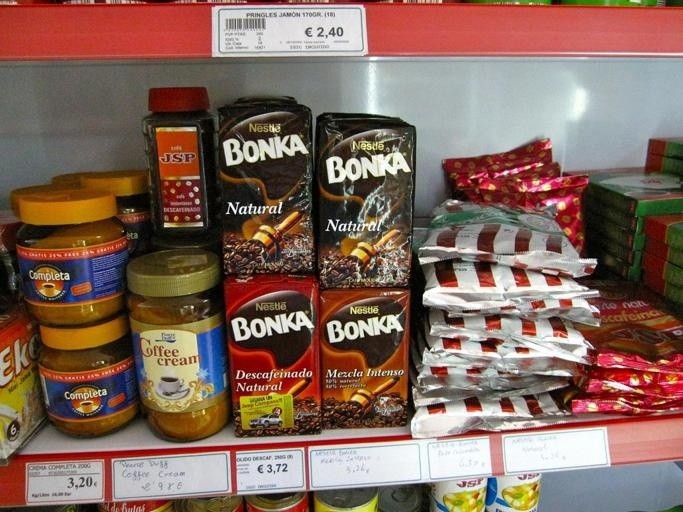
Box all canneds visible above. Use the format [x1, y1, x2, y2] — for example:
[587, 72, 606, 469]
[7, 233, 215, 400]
[314, 488, 379, 512]
[376, 483, 431, 512]
[431, 478, 489, 512]
[186, 495, 244, 512]
[11, 170, 233, 444]
[245, 490, 309, 512]
[486, 474, 542, 512]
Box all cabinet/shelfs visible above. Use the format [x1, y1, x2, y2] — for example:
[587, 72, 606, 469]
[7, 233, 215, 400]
[0, 0, 683, 511]
